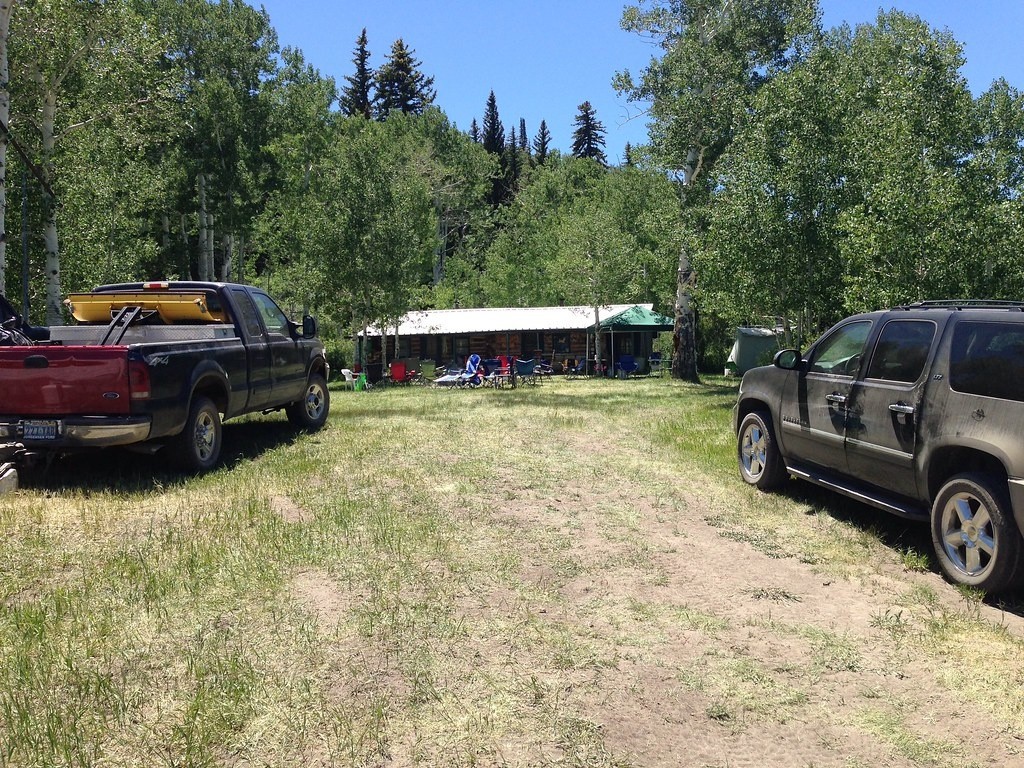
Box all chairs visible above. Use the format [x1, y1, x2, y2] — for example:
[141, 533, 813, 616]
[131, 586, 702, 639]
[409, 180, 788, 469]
[404, 351, 665, 388]
[886, 343, 931, 384]
[341, 369, 359, 391]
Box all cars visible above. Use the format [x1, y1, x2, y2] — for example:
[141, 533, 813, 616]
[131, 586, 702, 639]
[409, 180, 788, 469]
[731, 298, 1024, 596]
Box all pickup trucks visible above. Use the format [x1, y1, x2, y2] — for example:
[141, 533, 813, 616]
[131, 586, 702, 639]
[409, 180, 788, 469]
[0, 272, 334, 481]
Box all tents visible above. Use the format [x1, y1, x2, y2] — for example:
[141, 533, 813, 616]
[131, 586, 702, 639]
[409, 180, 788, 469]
[586, 305, 675, 380]
